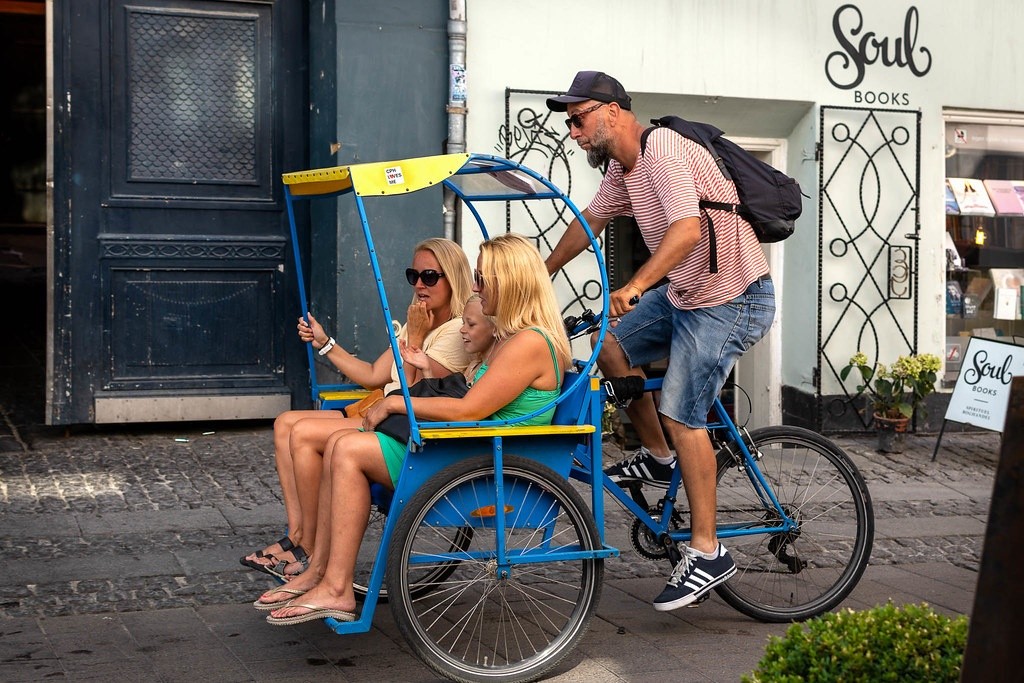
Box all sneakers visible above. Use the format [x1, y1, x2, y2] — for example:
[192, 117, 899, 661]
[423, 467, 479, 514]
[653, 542, 738, 612]
[602, 445, 683, 489]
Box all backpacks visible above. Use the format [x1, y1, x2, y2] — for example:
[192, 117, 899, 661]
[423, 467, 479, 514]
[640, 114, 811, 273]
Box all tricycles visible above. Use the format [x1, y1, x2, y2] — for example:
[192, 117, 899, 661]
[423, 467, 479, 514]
[273, 152, 875, 683]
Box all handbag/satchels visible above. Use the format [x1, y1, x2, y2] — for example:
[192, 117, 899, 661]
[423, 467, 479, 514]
[374, 372, 470, 445]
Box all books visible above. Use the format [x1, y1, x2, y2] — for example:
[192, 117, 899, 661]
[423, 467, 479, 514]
[946, 269, 1024, 321]
[945, 174, 1024, 223]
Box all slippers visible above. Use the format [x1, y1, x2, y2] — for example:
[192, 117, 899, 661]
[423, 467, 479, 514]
[266, 599, 356, 625]
[253, 585, 307, 610]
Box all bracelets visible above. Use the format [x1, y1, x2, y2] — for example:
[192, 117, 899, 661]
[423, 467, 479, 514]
[319, 337, 335, 355]
[318, 338, 330, 351]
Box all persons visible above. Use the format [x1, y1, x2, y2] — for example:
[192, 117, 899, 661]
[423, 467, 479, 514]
[238, 238, 474, 574]
[544, 72, 774, 611]
[253, 235, 569, 626]
[266, 294, 497, 583]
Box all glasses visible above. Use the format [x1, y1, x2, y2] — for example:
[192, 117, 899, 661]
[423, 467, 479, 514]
[565, 103, 604, 130]
[406, 268, 445, 287]
[474, 269, 497, 286]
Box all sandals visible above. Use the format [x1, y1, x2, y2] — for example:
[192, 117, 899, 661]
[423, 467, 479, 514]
[281, 576, 290, 584]
[248, 546, 308, 574]
[264, 556, 309, 578]
[240, 536, 295, 567]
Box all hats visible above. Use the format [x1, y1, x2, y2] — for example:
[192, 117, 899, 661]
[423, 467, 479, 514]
[546, 71, 632, 112]
